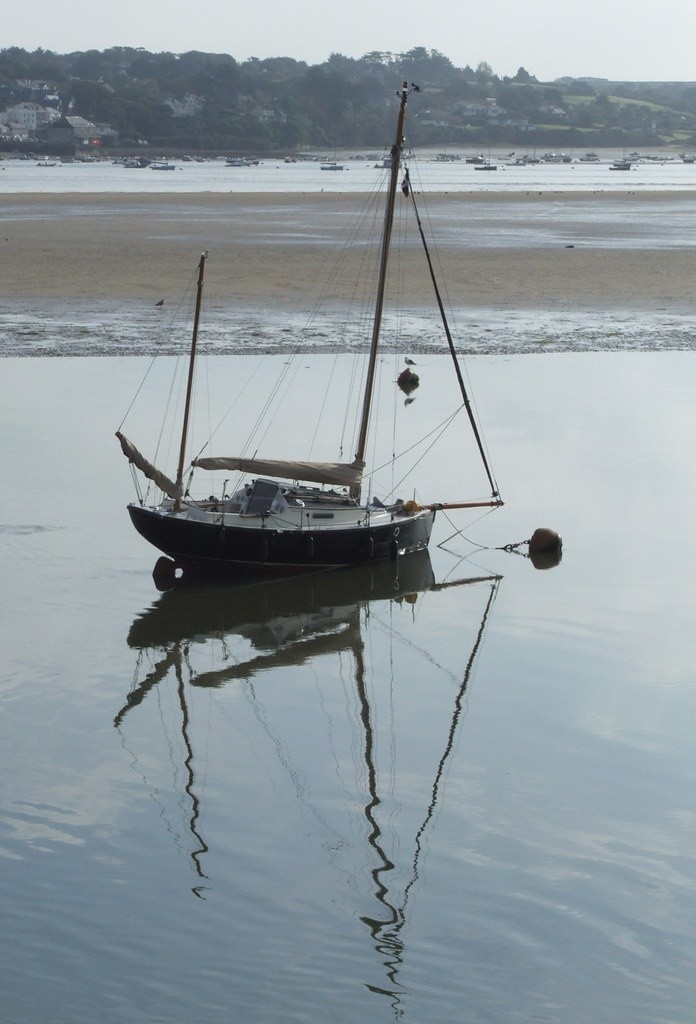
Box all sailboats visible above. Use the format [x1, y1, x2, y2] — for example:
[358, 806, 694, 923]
[113, 547, 505, 924]
[112, 74, 504, 566]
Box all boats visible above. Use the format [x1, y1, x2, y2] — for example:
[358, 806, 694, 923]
[36, 145, 696, 172]
